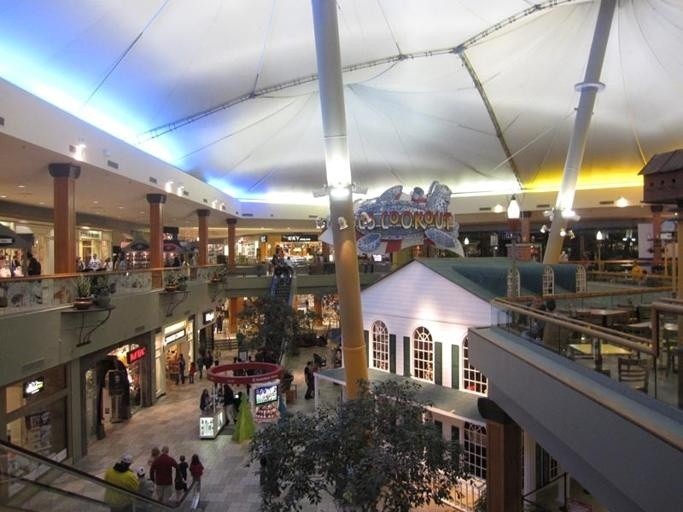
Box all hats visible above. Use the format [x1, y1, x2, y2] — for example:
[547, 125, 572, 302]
[137, 466, 146, 475]
[122, 455, 133, 463]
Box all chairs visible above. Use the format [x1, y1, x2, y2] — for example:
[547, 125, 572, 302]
[518, 303, 680, 394]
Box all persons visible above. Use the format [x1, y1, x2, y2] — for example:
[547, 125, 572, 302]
[270, 244, 294, 285]
[632, 260, 642, 281]
[304, 345, 343, 401]
[369, 254, 375, 271]
[104, 445, 205, 512]
[11, 251, 43, 278]
[179, 349, 254, 425]
[518, 297, 545, 336]
[642, 270, 648, 285]
[172, 247, 202, 278]
[76, 250, 127, 276]
[536, 300, 569, 336]
[364, 255, 369, 272]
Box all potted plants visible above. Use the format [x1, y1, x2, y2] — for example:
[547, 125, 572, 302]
[164, 272, 189, 291]
[72, 275, 111, 310]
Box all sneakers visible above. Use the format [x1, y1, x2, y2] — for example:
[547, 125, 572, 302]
[304, 394, 312, 399]
